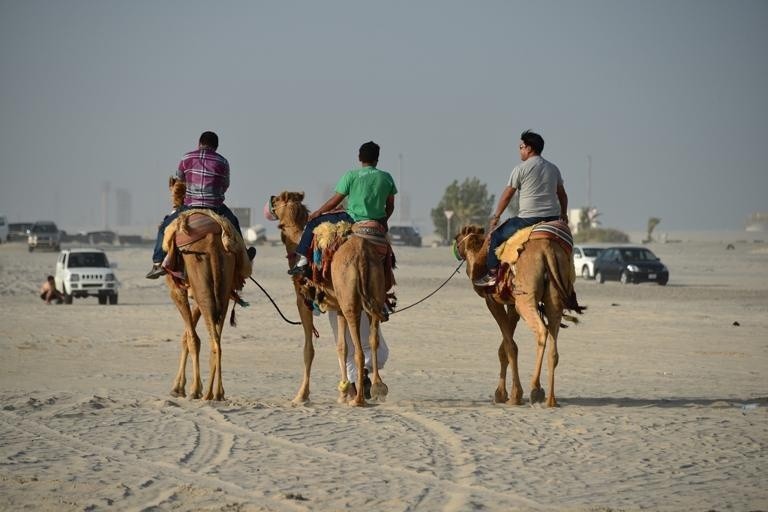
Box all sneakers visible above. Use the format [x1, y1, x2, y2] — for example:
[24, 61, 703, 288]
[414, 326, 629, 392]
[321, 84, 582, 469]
[146, 263, 167, 279]
[474, 270, 496, 286]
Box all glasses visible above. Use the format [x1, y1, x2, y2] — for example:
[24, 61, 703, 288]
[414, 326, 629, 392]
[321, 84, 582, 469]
[520, 145, 527, 150]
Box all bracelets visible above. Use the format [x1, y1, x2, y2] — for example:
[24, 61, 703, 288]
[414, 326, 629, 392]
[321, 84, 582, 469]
[493, 214, 501, 221]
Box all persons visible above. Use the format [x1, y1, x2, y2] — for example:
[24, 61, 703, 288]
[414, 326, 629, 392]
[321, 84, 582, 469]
[482, 131, 570, 275]
[38, 273, 66, 305]
[287, 141, 399, 276]
[146, 130, 256, 280]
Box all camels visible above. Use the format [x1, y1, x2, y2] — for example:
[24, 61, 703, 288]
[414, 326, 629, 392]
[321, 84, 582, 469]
[262, 190, 397, 407]
[165, 175, 250, 405]
[454, 220, 587, 409]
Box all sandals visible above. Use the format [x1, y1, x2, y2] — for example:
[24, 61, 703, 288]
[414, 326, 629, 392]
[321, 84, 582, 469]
[288, 264, 309, 275]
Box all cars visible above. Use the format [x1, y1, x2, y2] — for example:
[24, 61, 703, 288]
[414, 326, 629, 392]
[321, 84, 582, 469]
[573, 245, 669, 286]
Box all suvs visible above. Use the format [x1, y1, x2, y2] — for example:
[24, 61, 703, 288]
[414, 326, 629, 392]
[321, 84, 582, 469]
[387, 226, 421, 247]
[25, 221, 61, 253]
[53, 248, 118, 305]
